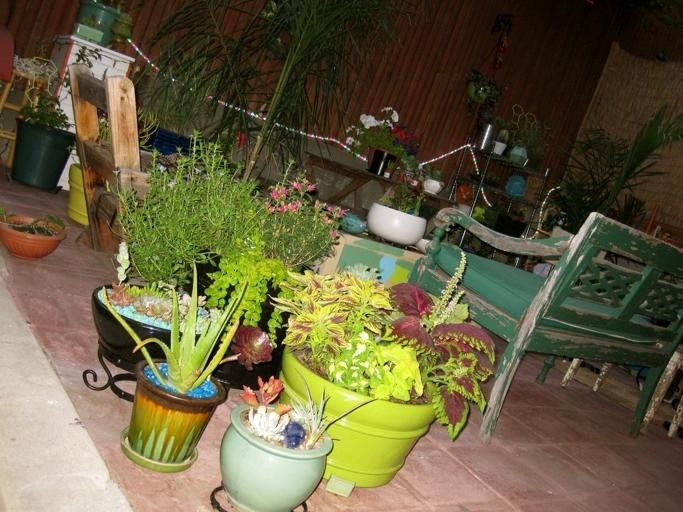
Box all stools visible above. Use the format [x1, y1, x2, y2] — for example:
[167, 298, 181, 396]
[560, 344, 683, 440]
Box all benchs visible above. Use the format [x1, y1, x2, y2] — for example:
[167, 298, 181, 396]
[408, 195, 683, 446]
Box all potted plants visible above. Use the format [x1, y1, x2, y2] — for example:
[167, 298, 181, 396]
[83, 129, 494, 505]
[64, 104, 161, 229]
[0, 207, 62, 263]
[462, 67, 503, 119]
[12, 92, 78, 194]
[345, 107, 406, 179]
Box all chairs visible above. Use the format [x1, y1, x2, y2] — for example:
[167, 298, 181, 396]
[69, 64, 178, 260]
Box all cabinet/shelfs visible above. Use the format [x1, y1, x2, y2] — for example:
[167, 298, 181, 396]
[449, 136, 550, 270]
